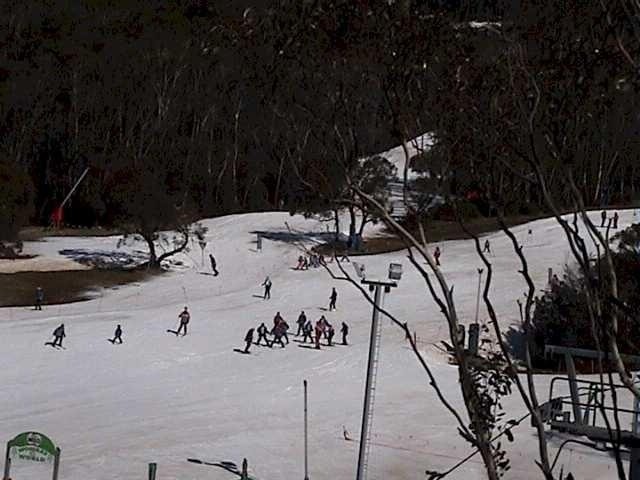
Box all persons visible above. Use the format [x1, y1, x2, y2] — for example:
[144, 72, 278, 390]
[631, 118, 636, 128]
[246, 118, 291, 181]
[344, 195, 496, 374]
[612, 212, 619, 229]
[295, 248, 350, 270]
[242, 312, 349, 353]
[53, 324, 66, 347]
[483, 239, 491, 253]
[208, 253, 219, 277]
[241, 458, 249, 480]
[261, 275, 272, 300]
[328, 287, 338, 311]
[111, 324, 123, 344]
[433, 247, 442, 268]
[177, 307, 190, 336]
[600, 210, 607, 227]
[34, 284, 43, 310]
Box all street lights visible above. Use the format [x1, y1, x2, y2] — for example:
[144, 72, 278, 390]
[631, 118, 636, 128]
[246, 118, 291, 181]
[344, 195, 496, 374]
[474, 267, 485, 322]
[351, 261, 406, 480]
